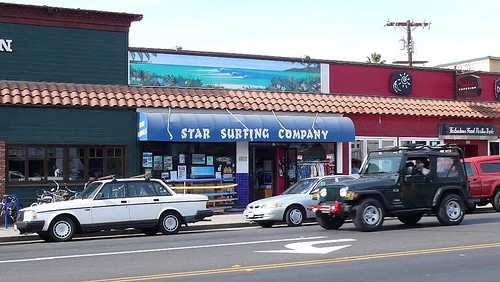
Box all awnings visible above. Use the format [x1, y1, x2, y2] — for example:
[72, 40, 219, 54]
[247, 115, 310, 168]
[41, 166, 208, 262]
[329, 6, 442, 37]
[136, 106, 355, 142]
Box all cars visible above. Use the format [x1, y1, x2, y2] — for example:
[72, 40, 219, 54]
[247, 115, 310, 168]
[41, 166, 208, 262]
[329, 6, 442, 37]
[242, 175, 359, 228]
[15, 178, 213, 244]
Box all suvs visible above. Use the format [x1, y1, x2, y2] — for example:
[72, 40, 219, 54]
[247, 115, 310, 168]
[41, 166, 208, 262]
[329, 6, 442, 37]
[315, 144, 475, 233]
[438, 155, 500, 214]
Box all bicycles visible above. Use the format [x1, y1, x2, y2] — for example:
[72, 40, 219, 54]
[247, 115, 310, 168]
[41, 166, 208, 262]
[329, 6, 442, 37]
[28, 175, 126, 211]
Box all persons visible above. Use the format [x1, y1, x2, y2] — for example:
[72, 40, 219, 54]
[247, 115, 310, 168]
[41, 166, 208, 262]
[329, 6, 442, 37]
[414, 158, 431, 176]
